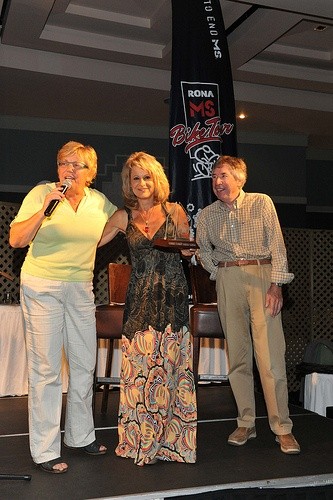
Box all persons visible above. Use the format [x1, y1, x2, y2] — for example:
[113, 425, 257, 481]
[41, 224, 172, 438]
[9, 142, 120, 474]
[195, 156, 300, 454]
[97, 153, 198, 465]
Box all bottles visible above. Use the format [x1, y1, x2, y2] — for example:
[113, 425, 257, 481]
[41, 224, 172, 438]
[4, 290, 12, 304]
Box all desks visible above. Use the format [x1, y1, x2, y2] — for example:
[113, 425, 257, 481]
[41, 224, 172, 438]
[0, 302, 229, 398]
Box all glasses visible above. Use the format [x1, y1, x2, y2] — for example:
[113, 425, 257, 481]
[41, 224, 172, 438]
[57, 161, 88, 169]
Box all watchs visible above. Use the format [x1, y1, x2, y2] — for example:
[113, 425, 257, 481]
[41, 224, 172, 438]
[272, 282, 283, 287]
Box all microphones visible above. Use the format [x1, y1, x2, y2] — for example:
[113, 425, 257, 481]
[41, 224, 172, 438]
[44, 179, 72, 217]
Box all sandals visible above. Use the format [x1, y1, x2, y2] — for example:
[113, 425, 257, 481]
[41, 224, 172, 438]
[39, 457, 68, 474]
[64, 439, 108, 455]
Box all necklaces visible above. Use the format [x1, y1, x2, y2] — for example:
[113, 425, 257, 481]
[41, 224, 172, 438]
[140, 211, 152, 233]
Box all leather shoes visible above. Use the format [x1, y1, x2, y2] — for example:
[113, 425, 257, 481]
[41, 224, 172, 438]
[228, 427, 256, 446]
[276, 433, 301, 455]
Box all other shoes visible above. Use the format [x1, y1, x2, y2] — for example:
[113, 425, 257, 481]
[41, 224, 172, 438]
[145, 459, 158, 464]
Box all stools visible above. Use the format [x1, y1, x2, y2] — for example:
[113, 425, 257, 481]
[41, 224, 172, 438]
[188, 264, 228, 392]
[91, 262, 133, 412]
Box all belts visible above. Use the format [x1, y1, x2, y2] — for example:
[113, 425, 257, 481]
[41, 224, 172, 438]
[218, 259, 271, 267]
